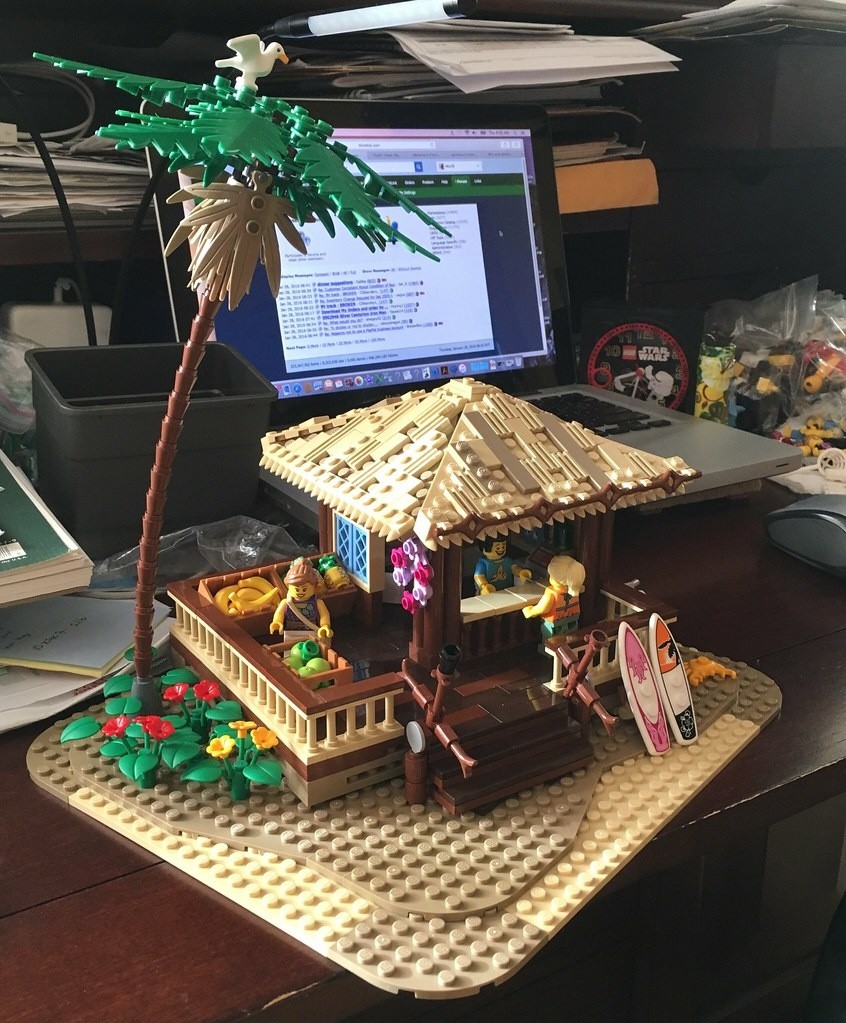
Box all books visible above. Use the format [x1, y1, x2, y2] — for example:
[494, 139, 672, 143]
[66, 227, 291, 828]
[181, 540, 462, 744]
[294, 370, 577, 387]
[0, 142, 154, 219]
[260, 18, 604, 104]
[626, 0, 846, 44]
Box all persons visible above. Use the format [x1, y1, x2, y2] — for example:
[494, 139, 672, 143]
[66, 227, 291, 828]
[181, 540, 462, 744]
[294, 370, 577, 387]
[472, 534, 533, 594]
[521, 556, 586, 671]
[0, 449, 172, 679]
[269, 560, 334, 639]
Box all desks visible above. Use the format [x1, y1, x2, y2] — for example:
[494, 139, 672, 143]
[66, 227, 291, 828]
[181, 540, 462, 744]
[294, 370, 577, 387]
[0, 476, 846, 1023]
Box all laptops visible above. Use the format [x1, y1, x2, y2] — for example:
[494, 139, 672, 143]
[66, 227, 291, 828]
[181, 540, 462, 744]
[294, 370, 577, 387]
[177, 98, 803, 507]
